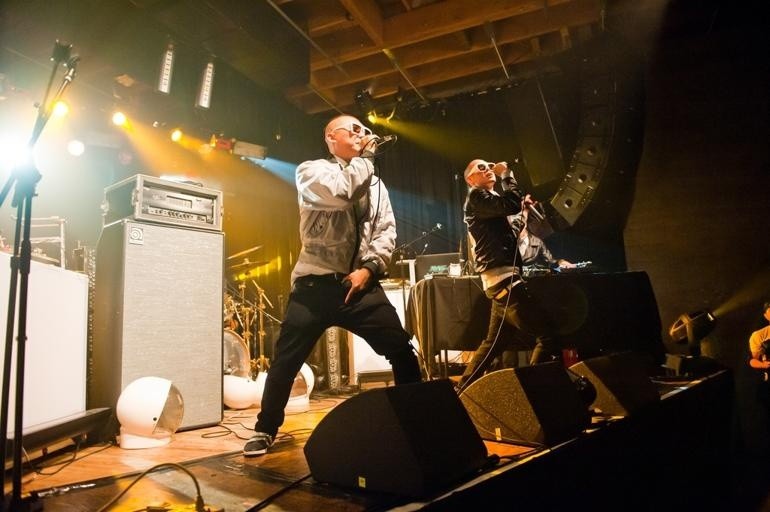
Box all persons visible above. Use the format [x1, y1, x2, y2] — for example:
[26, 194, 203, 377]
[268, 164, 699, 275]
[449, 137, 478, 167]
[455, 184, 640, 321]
[451, 158, 555, 391]
[243, 115, 422, 455]
[747, 302, 770, 382]
[509, 208, 577, 269]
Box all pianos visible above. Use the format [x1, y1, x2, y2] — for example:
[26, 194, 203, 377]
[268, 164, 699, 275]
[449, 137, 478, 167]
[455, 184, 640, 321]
[520, 262, 593, 278]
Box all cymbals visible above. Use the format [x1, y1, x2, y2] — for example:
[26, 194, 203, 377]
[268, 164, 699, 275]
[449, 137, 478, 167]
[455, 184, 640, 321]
[225, 245, 262, 261]
[225, 260, 271, 273]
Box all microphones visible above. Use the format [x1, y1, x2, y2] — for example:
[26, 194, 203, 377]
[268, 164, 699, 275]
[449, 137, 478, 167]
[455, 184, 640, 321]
[505, 159, 519, 168]
[519, 189, 545, 223]
[374, 135, 395, 145]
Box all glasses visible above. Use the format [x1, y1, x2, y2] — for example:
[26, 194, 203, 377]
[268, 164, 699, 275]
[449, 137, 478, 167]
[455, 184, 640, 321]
[332, 122, 373, 138]
[466, 162, 495, 180]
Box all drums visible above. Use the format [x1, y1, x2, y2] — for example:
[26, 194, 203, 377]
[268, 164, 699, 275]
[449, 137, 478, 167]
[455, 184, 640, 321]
[223, 329, 251, 378]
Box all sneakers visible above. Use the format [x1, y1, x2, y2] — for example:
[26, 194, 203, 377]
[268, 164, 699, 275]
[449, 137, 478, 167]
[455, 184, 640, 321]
[243, 432, 274, 456]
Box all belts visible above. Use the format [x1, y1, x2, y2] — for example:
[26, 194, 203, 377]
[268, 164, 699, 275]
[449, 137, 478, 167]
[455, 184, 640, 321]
[317, 272, 347, 279]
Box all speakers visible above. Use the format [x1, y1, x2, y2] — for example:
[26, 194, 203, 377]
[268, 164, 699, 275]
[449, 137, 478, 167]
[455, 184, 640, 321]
[458, 359, 572, 446]
[569, 351, 660, 418]
[303, 378, 488, 501]
[90, 220, 224, 434]
[549, 34, 646, 234]
